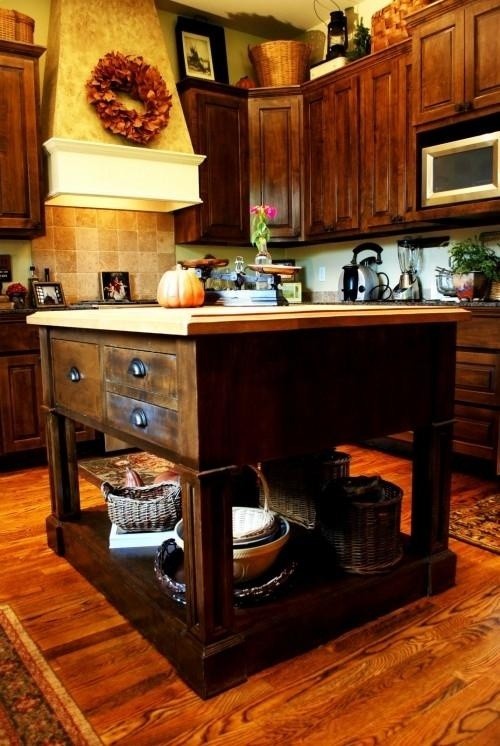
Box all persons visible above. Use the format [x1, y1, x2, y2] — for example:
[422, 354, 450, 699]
[104, 275, 129, 302]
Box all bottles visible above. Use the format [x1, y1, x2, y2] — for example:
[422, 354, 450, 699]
[28, 264, 39, 308]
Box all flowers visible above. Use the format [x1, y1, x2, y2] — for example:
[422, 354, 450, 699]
[83, 51, 176, 147]
[245, 203, 278, 249]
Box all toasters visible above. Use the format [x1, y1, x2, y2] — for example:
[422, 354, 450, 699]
[338, 241, 381, 303]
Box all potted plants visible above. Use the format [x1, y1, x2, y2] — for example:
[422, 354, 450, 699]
[448, 237, 500, 301]
[347, 15, 370, 59]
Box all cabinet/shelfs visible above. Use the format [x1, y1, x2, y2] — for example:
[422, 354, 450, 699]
[1, 322, 96, 453]
[412, 0, 500, 126]
[197, 88, 305, 240]
[452, 309, 500, 460]
[37, 320, 465, 704]
[305, 51, 413, 235]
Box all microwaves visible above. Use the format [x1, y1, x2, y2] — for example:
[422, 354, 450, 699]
[420, 134, 499, 206]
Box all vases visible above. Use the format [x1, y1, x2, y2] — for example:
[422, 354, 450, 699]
[254, 243, 271, 282]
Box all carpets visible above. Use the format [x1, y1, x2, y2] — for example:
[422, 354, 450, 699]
[441, 491, 500, 557]
[74, 450, 176, 494]
[0, 604, 110, 746]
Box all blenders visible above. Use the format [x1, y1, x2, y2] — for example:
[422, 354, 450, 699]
[394, 238, 421, 300]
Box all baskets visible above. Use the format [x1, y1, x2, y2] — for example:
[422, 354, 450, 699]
[101, 482, 182, 533]
[232, 464, 275, 538]
[259, 452, 352, 530]
[321, 476, 404, 574]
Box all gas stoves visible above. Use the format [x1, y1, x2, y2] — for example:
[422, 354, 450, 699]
[72, 299, 160, 309]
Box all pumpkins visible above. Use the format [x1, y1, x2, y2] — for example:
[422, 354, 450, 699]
[122, 466, 144, 487]
[157, 263, 205, 307]
[153, 470, 179, 484]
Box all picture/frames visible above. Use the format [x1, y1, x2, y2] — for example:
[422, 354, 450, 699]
[32, 281, 67, 308]
[175, 15, 230, 84]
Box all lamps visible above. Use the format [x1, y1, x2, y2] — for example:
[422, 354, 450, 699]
[325, 11, 349, 61]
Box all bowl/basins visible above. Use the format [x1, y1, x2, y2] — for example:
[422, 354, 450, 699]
[172, 516, 289, 583]
[436, 274, 462, 297]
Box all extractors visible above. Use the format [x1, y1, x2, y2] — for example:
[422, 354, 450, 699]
[41, 0, 207, 214]
